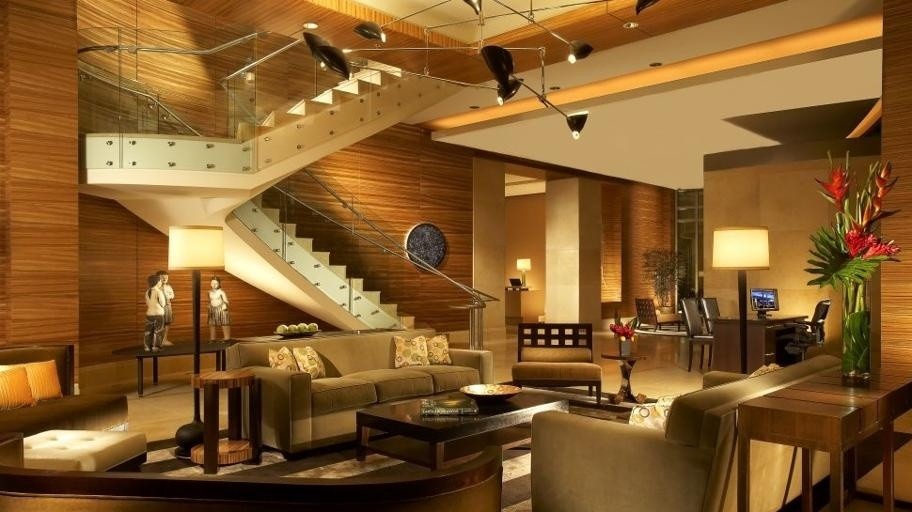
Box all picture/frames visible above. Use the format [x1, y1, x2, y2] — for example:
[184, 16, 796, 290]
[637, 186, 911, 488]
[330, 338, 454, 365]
[405, 223, 447, 272]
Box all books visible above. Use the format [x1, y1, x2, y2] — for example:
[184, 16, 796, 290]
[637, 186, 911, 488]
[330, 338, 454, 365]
[419, 397, 478, 416]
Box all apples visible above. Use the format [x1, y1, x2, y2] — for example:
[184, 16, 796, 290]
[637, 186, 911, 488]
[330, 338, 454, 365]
[289, 324, 300, 333]
[276, 325, 288, 334]
[298, 323, 308, 334]
[308, 323, 318, 332]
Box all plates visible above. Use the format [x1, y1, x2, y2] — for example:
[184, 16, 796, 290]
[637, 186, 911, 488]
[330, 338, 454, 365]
[273, 330, 321, 337]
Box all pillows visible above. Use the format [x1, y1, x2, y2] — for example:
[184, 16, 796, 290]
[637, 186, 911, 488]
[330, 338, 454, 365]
[656, 397, 675, 403]
[749, 363, 784, 378]
[629, 403, 671, 430]
[292, 346, 326, 379]
[227, 343, 269, 369]
[394, 335, 430, 368]
[0, 359, 64, 401]
[0, 366, 38, 413]
[426, 335, 452, 365]
[269, 346, 301, 372]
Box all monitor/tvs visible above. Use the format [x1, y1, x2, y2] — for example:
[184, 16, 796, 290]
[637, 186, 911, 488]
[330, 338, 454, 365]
[750, 288, 779, 319]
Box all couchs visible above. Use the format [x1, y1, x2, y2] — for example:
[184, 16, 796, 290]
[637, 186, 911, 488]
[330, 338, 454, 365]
[226, 329, 493, 460]
[23, 428, 147, 472]
[512, 323, 601, 403]
[0, 343, 129, 431]
[531, 354, 842, 512]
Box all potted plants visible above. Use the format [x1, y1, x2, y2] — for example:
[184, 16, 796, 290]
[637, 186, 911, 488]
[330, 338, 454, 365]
[641, 247, 681, 325]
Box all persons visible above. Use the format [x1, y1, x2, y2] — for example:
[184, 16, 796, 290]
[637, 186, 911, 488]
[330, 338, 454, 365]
[206, 276, 231, 345]
[155, 271, 174, 348]
[144, 276, 166, 352]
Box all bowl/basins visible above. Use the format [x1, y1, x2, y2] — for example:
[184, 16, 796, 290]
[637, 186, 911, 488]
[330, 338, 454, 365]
[459, 383, 524, 403]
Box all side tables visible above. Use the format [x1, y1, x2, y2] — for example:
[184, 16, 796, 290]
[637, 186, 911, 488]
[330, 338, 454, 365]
[191, 371, 263, 474]
[601, 353, 649, 404]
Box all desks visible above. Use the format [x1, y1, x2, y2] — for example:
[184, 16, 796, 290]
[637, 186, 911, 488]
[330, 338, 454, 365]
[111, 340, 239, 396]
[737, 368, 912, 512]
[505, 286, 545, 325]
[707, 318, 809, 374]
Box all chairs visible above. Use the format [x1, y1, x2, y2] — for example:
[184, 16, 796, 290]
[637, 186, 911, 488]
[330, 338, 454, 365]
[776, 300, 830, 360]
[635, 298, 682, 332]
[680, 299, 713, 372]
[700, 298, 720, 334]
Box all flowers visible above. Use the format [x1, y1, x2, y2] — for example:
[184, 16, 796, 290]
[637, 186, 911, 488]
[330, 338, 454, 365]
[802, 149, 902, 377]
[609, 309, 638, 342]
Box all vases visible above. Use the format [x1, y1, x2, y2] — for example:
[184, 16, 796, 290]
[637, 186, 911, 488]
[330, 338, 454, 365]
[619, 339, 630, 357]
[842, 278, 873, 381]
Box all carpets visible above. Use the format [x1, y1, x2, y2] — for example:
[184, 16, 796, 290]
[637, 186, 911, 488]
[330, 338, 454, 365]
[142, 382, 658, 512]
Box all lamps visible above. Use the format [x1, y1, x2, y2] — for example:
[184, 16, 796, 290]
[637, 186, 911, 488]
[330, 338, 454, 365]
[303, 0, 660, 139]
[168, 225, 225, 460]
[516, 258, 531, 291]
[713, 226, 770, 374]
